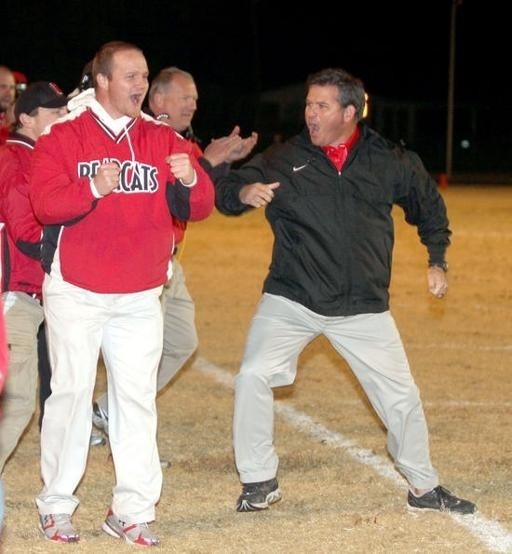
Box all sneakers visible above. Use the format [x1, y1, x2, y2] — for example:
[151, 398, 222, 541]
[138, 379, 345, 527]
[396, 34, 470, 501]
[236, 478, 282, 512]
[101, 506, 161, 547]
[38, 513, 81, 543]
[407, 485, 479, 516]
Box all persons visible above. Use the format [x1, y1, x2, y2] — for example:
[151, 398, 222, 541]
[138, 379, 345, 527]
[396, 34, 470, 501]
[2, 64, 261, 489]
[28, 38, 217, 546]
[206, 67, 483, 517]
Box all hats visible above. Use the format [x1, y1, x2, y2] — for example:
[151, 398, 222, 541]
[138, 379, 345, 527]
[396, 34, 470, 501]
[14, 81, 71, 122]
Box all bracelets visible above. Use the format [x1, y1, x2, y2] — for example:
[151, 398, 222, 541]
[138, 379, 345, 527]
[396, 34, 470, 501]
[427, 261, 448, 273]
